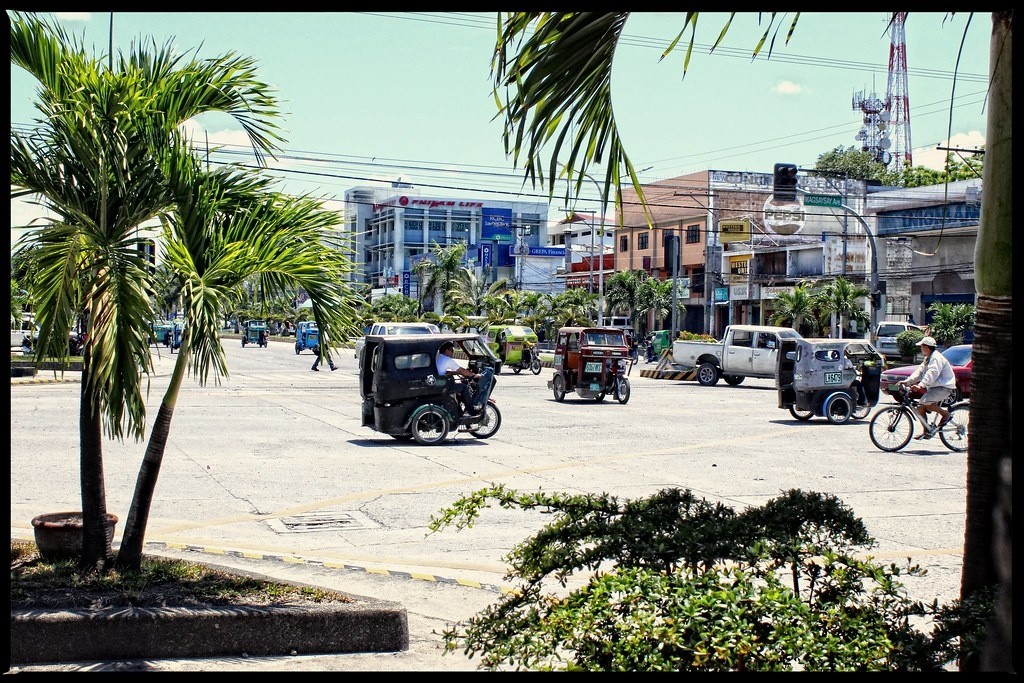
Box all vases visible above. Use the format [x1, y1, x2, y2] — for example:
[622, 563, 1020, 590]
[30, 512, 119, 561]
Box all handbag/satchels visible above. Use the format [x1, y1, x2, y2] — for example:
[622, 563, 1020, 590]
[313, 343, 320, 356]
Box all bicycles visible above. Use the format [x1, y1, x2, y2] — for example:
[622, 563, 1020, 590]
[869, 384, 969, 453]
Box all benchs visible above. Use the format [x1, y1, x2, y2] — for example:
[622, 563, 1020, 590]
[568, 351, 578, 372]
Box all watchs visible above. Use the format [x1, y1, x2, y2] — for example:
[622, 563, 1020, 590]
[848, 317, 858, 339]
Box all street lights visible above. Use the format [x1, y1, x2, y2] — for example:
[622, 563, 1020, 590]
[566, 247, 593, 295]
[315, 229, 378, 298]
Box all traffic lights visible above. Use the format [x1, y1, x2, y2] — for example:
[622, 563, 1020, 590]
[773, 163, 798, 201]
[870, 291, 880, 310]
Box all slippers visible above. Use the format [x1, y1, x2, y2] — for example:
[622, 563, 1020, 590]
[938, 416, 953, 426]
[914, 433, 931, 439]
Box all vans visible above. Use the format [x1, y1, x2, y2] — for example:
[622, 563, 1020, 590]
[874, 322, 926, 365]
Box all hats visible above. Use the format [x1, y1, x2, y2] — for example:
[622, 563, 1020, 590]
[915, 337, 938, 347]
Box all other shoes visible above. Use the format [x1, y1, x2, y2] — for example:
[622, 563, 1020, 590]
[463, 411, 471, 417]
[469, 413, 482, 418]
[312, 366, 319, 371]
[332, 367, 338, 371]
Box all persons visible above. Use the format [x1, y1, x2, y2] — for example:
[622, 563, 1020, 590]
[908, 314, 915, 324]
[894, 337, 958, 440]
[423, 341, 485, 418]
[830, 350, 870, 407]
[311, 328, 339, 372]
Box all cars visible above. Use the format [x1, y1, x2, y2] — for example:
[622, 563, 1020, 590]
[429, 324, 440, 334]
[10, 314, 84, 355]
[280, 322, 295, 334]
[879, 344, 973, 407]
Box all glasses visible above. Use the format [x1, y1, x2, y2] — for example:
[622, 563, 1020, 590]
[448, 348, 453, 353]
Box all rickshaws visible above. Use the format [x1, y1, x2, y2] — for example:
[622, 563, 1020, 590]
[295, 322, 324, 356]
[775, 336, 886, 425]
[170, 323, 185, 354]
[548, 324, 635, 403]
[644, 330, 680, 363]
[359, 333, 502, 446]
[602, 325, 639, 365]
[241, 319, 269, 348]
[151, 320, 172, 347]
[485, 325, 542, 375]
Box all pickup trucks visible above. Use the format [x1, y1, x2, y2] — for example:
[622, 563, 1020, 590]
[354, 322, 440, 361]
[670, 324, 804, 387]
